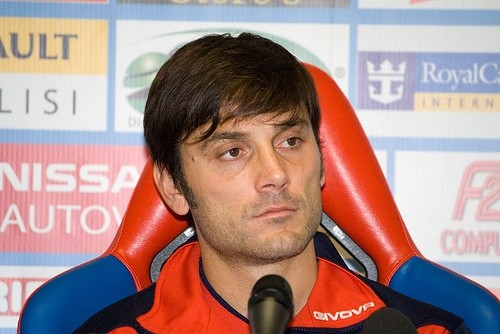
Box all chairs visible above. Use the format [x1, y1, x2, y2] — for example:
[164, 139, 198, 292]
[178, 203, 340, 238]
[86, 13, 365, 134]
[16, 60, 500, 334]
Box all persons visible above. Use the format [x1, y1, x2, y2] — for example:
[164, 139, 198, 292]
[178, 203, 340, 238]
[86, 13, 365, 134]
[72, 32, 471, 333]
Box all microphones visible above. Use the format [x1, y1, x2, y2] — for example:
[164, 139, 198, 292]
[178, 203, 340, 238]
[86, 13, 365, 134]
[361, 307, 419, 334]
[247, 275, 293, 334]
